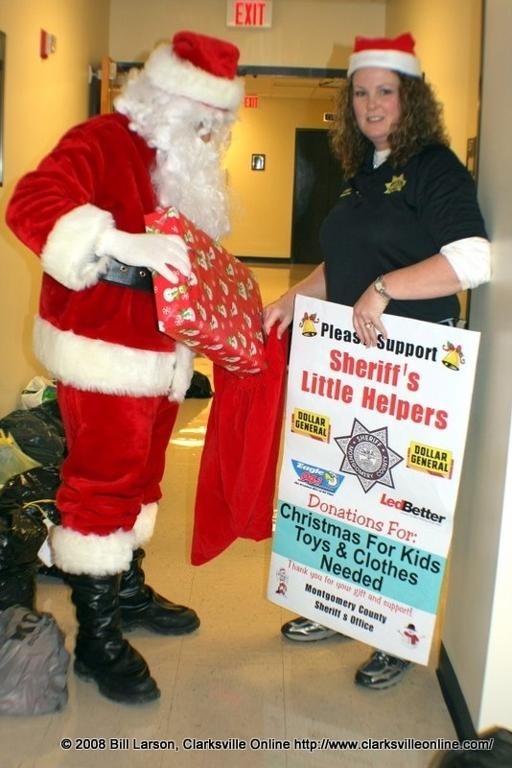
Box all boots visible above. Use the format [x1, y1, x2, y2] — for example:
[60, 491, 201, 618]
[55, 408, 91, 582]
[72, 547, 200, 704]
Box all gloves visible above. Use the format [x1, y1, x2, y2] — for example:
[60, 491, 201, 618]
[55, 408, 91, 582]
[94, 227, 192, 284]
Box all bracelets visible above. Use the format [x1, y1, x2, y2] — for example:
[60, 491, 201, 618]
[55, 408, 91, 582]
[374, 272, 391, 300]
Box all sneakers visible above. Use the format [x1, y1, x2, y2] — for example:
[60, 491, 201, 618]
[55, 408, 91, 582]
[281, 615, 337, 643]
[355, 650, 411, 690]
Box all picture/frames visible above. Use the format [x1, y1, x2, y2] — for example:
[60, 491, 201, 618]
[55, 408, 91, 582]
[252, 154, 265, 170]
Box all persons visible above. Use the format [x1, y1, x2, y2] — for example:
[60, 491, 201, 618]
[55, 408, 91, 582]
[6, 32, 244, 703]
[258, 32, 494, 690]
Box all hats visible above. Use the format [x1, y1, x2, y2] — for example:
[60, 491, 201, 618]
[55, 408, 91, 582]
[348, 32, 422, 79]
[144, 29, 245, 111]
